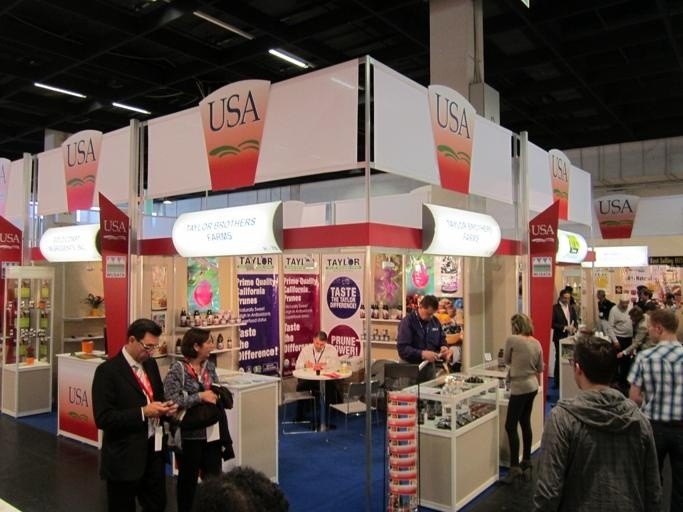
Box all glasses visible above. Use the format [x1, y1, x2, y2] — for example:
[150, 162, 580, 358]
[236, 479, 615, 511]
[139, 340, 161, 351]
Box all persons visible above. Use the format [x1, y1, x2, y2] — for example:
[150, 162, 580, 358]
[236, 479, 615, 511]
[503, 313, 545, 484]
[179, 465, 289, 512]
[91, 318, 179, 511]
[294, 330, 342, 430]
[397, 295, 449, 382]
[162, 326, 235, 512]
[436, 299, 463, 373]
[406, 293, 421, 316]
[530, 284, 683, 512]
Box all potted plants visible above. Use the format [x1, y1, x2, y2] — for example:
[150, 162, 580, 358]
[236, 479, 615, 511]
[81, 294, 104, 316]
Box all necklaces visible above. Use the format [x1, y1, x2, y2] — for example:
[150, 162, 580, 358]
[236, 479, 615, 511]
[312, 348, 325, 365]
[130, 364, 164, 452]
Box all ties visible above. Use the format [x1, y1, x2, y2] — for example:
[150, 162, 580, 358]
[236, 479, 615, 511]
[134, 364, 147, 399]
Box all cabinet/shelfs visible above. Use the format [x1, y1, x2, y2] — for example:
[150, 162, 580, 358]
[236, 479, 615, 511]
[559, 335, 611, 402]
[404, 374, 499, 512]
[471, 358, 543, 468]
[0, 267, 54, 418]
[354, 317, 400, 345]
[64, 317, 106, 343]
[172, 324, 248, 359]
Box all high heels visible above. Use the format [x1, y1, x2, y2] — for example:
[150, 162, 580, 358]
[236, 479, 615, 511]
[495, 461, 533, 485]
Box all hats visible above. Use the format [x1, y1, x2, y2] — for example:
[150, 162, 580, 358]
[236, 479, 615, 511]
[597, 284, 648, 301]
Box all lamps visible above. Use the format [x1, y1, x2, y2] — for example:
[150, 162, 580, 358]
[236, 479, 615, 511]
[172, 202, 284, 258]
[554, 230, 587, 265]
[39, 226, 102, 264]
[422, 201, 502, 258]
[581, 245, 648, 268]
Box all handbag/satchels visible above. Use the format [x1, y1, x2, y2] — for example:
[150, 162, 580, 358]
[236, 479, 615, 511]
[180, 393, 218, 432]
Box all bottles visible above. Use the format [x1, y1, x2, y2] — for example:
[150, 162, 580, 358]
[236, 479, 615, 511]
[226, 337, 231, 348]
[417, 392, 443, 426]
[369, 304, 402, 320]
[179, 309, 226, 326]
[359, 304, 364, 317]
[497, 348, 504, 367]
[193, 278, 216, 307]
[216, 334, 223, 349]
[175, 338, 181, 353]
[440, 256, 457, 292]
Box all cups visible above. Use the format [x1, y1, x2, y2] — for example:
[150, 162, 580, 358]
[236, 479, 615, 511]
[228, 318, 240, 323]
[80, 341, 94, 353]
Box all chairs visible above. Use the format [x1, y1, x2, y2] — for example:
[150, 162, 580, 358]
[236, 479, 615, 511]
[377, 365, 419, 418]
[277, 371, 317, 436]
[327, 381, 383, 450]
